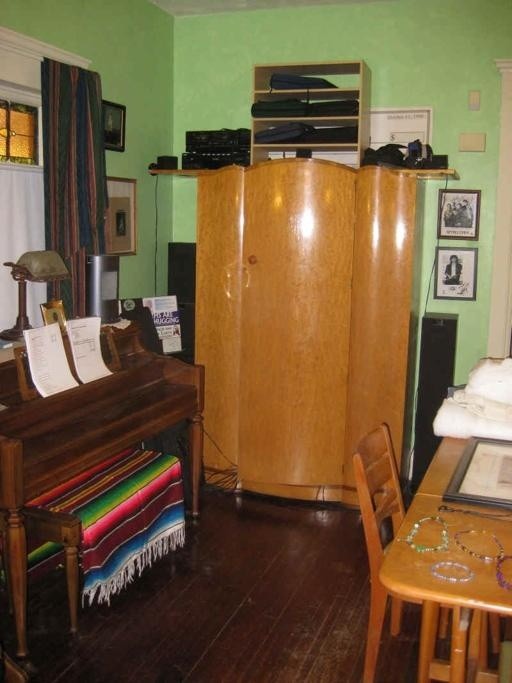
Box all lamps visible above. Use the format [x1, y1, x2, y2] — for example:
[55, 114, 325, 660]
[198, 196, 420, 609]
[0, 250, 70, 341]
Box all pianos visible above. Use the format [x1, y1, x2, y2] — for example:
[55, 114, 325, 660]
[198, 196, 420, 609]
[0, 317, 205, 668]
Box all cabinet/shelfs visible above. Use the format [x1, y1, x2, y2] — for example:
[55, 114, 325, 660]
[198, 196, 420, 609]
[195, 157, 417, 506]
[250, 59, 371, 168]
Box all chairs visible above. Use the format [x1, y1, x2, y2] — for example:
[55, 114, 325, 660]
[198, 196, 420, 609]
[351, 422, 500, 683]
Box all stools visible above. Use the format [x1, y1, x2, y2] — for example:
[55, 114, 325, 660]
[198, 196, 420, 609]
[20, 448, 187, 633]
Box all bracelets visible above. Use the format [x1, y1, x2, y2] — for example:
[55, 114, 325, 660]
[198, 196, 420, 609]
[431, 560, 474, 582]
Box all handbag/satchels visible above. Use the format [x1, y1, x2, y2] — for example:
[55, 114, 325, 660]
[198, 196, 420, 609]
[271, 75, 338, 88]
[251, 100, 308, 117]
[303, 127, 357, 143]
[310, 100, 359, 115]
[256, 122, 304, 143]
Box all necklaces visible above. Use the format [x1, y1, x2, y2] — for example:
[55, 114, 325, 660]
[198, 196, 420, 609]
[496, 556, 512, 591]
[406, 514, 450, 552]
[453, 529, 504, 561]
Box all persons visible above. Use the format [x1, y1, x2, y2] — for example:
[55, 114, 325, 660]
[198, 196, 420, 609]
[443, 199, 473, 228]
[444, 255, 463, 285]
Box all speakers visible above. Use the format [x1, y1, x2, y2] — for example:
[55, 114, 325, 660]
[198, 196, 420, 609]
[410, 310, 457, 497]
[168, 242, 196, 364]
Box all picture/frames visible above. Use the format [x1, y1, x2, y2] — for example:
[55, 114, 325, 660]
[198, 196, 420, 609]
[434, 246, 479, 302]
[443, 436, 512, 509]
[106, 176, 138, 256]
[437, 189, 481, 241]
[39, 300, 66, 326]
[102, 99, 126, 152]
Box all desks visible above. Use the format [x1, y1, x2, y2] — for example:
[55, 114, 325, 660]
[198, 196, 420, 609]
[380, 437, 512, 683]
